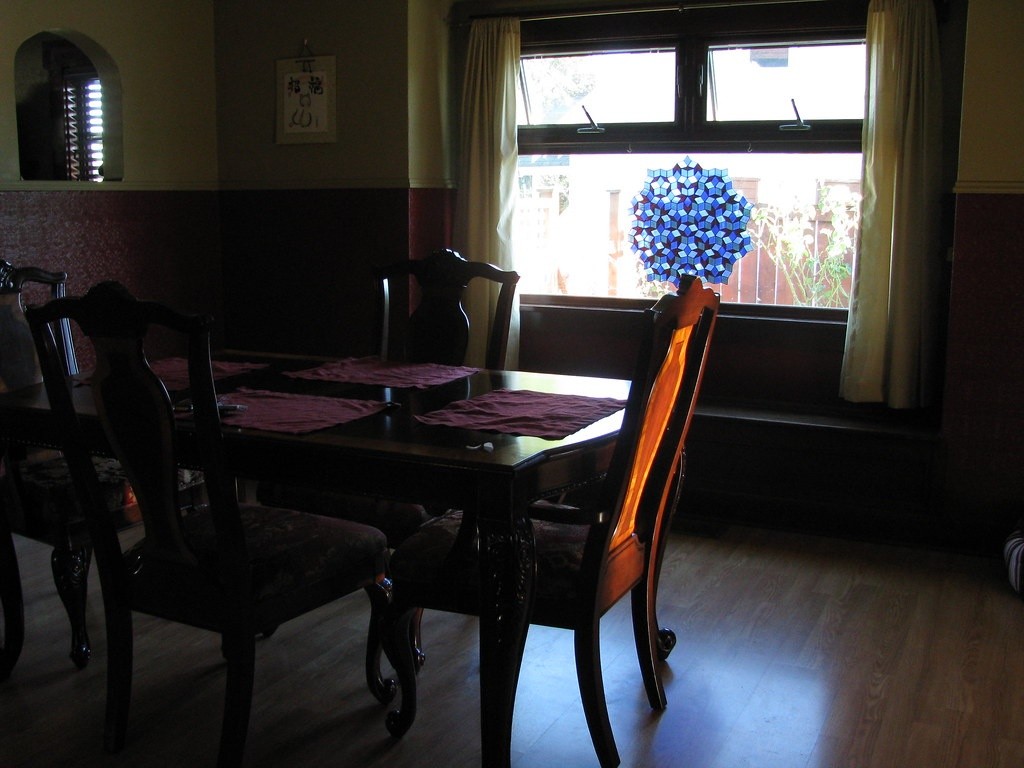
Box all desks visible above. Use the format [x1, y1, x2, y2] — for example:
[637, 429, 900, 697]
[0, 349, 633, 768]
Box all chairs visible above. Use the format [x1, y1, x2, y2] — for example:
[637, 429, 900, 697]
[0, 258, 206, 685]
[376, 273, 721, 768]
[372, 252, 512, 677]
[23, 280, 395, 768]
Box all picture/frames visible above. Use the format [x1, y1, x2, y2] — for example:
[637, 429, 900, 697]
[275, 55, 336, 145]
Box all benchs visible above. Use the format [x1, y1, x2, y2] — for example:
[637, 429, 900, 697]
[519, 303, 949, 548]
